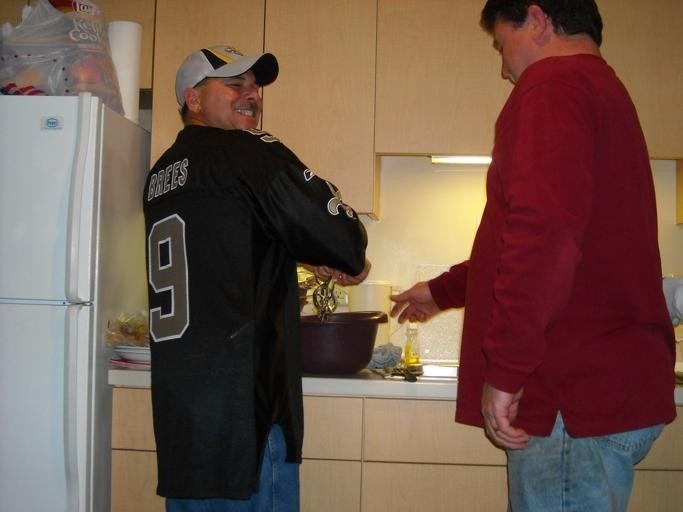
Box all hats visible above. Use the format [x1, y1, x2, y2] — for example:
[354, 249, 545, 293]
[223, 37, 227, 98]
[175, 44, 279, 107]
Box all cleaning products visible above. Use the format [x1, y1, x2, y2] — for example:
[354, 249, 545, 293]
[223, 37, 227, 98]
[406, 320, 420, 365]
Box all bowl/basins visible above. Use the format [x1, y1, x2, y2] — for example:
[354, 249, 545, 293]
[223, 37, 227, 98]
[300, 311, 388, 376]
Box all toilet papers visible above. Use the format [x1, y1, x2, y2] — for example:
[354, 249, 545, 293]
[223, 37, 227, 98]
[108, 22, 140, 124]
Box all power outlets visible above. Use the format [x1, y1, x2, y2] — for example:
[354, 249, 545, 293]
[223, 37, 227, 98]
[329, 285, 349, 304]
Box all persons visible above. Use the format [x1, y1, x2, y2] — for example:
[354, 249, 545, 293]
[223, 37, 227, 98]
[387, 0, 677, 511]
[142, 47, 372, 511]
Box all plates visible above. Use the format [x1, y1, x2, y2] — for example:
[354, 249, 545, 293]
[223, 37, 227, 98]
[109, 346, 151, 371]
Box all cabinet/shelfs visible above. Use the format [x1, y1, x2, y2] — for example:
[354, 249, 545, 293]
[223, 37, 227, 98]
[297, 395, 364, 512]
[150, 1, 265, 169]
[361, 396, 509, 512]
[375, 1, 682, 162]
[626, 405, 683, 512]
[0, 0, 155, 89]
[108, 390, 166, 512]
[265, 1, 380, 217]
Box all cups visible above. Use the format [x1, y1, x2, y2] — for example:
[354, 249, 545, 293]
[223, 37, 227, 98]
[347, 278, 408, 349]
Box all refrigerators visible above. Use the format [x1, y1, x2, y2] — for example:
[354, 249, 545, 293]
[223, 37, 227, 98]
[1, 91, 151, 512]
